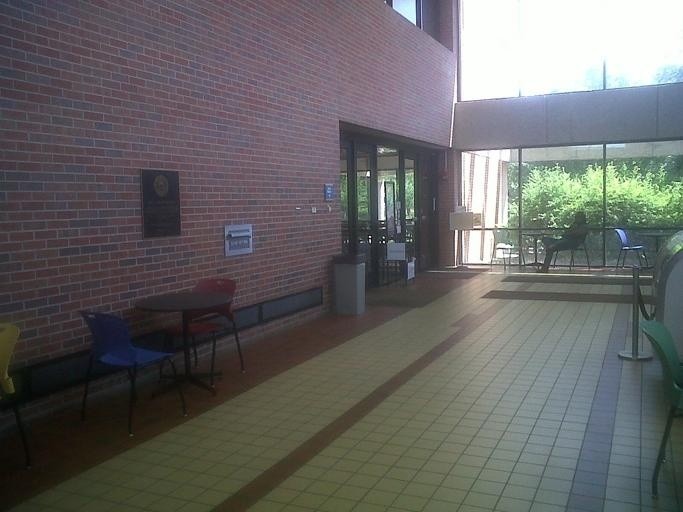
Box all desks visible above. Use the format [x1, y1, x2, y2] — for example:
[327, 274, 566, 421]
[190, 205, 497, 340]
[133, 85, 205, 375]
[135, 292, 232, 418]
[521, 232, 553, 270]
[636, 231, 673, 256]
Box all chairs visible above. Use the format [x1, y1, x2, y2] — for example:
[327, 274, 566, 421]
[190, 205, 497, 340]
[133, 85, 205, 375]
[639, 320, 683, 498]
[553, 229, 591, 271]
[0, 322, 33, 471]
[490, 224, 526, 274]
[76, 309, 188, 438]
[164, 277, 247, 389]
[613, 228, 649, 274]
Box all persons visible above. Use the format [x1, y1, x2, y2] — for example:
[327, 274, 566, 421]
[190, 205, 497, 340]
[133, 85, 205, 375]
[535, 212, 587, 272]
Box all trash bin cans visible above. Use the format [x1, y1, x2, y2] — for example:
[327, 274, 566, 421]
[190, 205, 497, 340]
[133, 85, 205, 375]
[332, 253, 366, 315]
